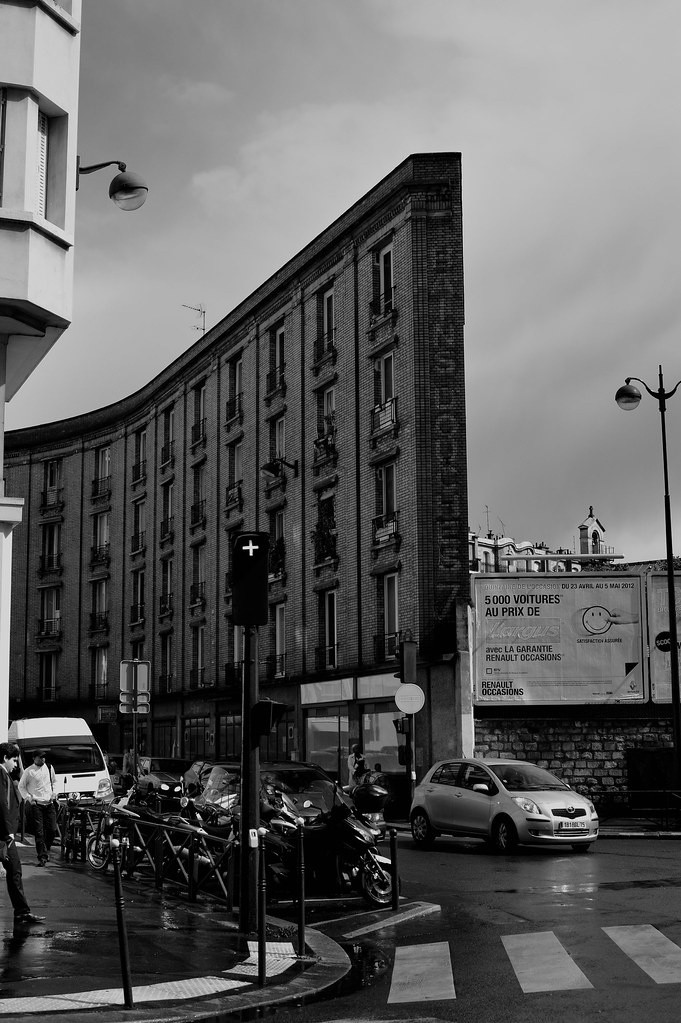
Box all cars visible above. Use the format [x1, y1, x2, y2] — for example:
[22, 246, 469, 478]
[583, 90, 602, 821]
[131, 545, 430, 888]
[409, 758, 600, 852]
[204, 764, 356, 830]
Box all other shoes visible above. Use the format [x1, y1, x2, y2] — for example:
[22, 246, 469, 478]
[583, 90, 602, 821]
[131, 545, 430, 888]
[14, 913, 46, 923]
[47, 855, 50, 862]
[37, 859, 46, 867]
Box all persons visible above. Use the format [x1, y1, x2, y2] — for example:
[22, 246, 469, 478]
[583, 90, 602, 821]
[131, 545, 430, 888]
[348, 745, 370, 797]
[18, 749, 57, 866]
[122, 745, 146, 777]
[0, 742, 46, 922]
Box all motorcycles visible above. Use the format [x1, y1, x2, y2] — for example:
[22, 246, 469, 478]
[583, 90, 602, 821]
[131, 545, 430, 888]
[58, 776, 401, 907]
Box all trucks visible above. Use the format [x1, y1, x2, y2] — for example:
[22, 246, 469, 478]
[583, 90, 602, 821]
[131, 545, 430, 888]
[9, 717, 115, 807]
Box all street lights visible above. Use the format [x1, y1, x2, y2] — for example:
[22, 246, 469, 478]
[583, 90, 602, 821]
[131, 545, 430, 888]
[614, 364, 681, 754]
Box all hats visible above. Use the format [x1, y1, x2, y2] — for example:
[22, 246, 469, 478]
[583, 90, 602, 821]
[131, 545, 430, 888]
[352, 743, 360, 752]
[33, 749, 46, 758]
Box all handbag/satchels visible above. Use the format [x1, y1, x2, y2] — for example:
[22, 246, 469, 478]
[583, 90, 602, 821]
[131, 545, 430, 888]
[0, 841, 10, 863]
